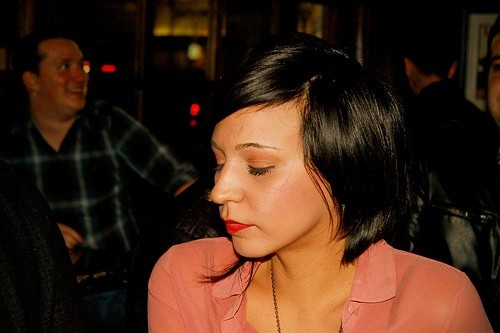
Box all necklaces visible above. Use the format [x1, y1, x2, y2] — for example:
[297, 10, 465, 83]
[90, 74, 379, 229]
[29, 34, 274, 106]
[268, 258, 347, 333]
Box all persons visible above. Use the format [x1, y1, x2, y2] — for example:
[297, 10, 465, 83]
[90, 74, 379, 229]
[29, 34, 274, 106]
[0, 25, 203, 333]
[396, 13, 500, 333]
[396, 21, 498, 185]
[0, 68, 90, 332]
[146, 34, 494, 333]
